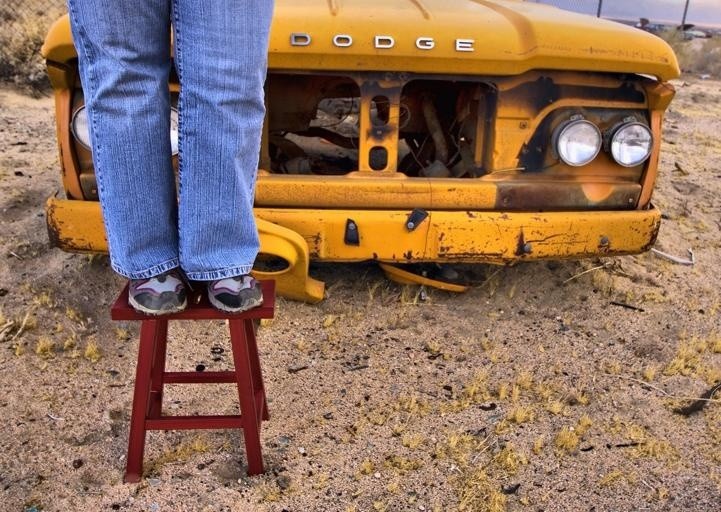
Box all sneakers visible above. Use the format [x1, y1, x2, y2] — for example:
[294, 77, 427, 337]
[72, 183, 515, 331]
[205, 271, 264, 316]
[127, 266, 197, 318]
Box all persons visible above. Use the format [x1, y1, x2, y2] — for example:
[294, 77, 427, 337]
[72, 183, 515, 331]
[67, 0, 274, 318]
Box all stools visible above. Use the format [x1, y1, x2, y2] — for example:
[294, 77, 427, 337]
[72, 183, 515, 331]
[112, 276, 276, 483]
